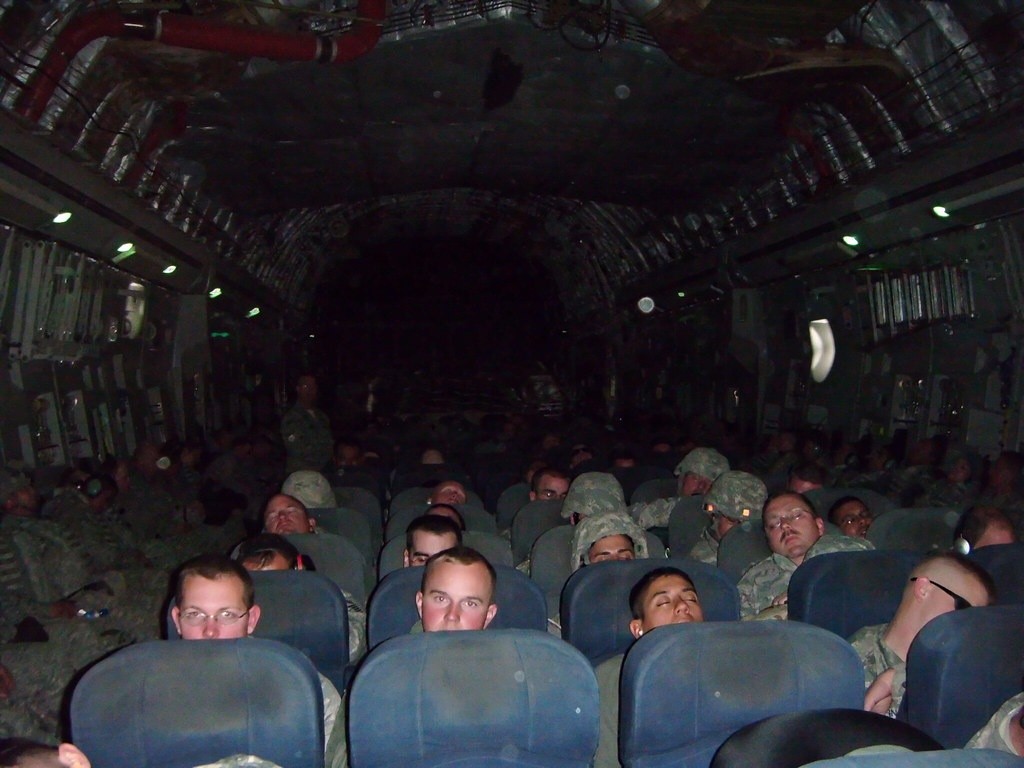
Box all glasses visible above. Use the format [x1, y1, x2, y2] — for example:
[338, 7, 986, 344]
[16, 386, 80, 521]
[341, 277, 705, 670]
[535, 490, 567, 500]
[758, 508, 815, 532]
[909, 576, 973, 611]
[841, 510, 872, 530]
[177, 607, 249, 625]
[717, 510, 741, 525]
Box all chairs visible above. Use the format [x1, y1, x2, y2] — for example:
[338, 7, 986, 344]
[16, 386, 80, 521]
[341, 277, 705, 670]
[72, 441, 1024, 768]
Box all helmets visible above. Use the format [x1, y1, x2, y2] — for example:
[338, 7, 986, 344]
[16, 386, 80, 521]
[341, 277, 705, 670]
[569, 511, 649, 575]
[280, 469, 337, 510]
[0, 467, 31, 508]
[700, 471, 768, 520]
[560, 471, 628, 519]
[672, 445, 730, 485]
[46, 463, 73, 491]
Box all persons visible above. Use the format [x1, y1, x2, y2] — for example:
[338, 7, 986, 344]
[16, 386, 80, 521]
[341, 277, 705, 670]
[0, 369, 1024, 768]
